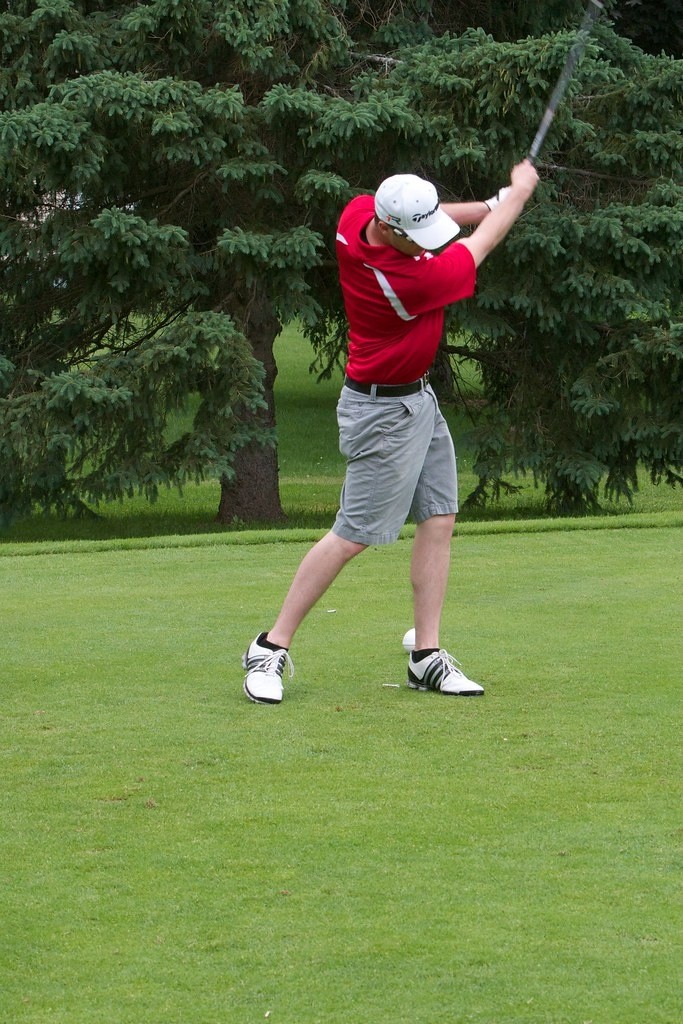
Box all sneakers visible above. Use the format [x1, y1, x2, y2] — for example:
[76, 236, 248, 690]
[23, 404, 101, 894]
[241, 632, 294, 704]
[409, 649, 484, 695]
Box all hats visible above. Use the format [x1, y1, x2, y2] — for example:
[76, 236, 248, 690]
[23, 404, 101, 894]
[375, 174, 460, 250]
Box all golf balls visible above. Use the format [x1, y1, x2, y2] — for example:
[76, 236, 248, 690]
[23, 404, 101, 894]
[403, 627, 415, 651]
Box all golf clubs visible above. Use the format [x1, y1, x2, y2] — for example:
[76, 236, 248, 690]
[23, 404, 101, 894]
[529, 0, 607, 159]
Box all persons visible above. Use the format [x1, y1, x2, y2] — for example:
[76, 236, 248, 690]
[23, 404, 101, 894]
[242, 159, 538, 704]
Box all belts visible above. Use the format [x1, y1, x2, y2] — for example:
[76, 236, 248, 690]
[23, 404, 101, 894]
[345, 371, 429, 397]
